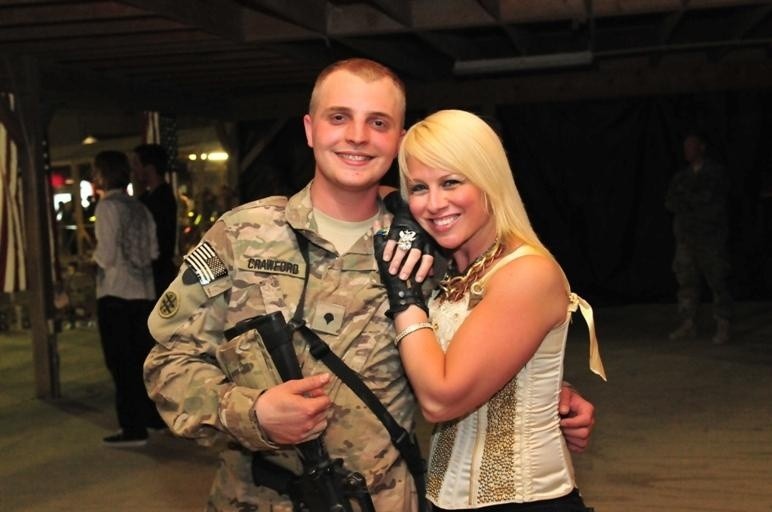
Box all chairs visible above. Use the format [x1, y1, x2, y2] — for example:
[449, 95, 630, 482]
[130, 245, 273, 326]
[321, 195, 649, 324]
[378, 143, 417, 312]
[101, 429, 149, 450]
[148, 418, 169, 434]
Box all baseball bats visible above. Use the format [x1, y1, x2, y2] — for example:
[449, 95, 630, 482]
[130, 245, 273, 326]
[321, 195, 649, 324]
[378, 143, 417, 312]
[249, 310, 375, 512]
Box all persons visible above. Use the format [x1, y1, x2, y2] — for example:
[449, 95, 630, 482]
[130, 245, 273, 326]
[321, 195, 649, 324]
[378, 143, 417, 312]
[59, 183, 231, 254]
[66, 149, 163, 450]
[141, 54, 596, 511]
[129, 142, 175, 293]
[374, 107, 594, 512]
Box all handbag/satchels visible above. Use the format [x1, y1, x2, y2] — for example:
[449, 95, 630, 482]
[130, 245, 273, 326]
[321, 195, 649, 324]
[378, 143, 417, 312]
[389, 322, 435, 347]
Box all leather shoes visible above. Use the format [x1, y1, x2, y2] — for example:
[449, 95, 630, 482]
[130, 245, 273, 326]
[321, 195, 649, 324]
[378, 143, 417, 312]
[383, 190, 435, 256]
[373, 233, 429, 318]
